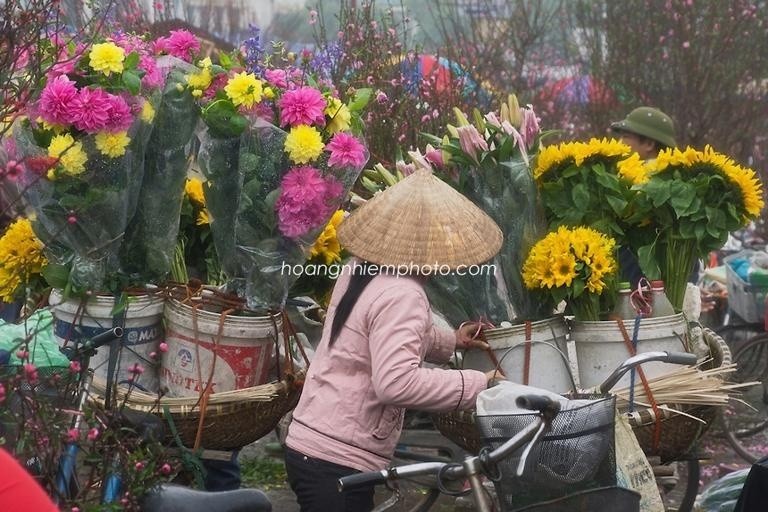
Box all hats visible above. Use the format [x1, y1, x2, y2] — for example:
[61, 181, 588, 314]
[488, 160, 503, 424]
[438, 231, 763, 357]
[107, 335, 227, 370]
[610, 106, 676, 150]
[335, 167, 507, 275]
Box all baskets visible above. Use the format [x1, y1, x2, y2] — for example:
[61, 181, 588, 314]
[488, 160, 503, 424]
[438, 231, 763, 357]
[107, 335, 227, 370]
[723, 248, 768, 324]
[77, 374, 307, 452]
[431, 325, 733, 457]
[473, 392, 619, 507]
[15, 364, 73, 410]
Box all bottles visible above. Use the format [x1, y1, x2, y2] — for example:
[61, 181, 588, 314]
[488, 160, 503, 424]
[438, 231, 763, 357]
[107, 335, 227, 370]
[612, 280, 676, 320]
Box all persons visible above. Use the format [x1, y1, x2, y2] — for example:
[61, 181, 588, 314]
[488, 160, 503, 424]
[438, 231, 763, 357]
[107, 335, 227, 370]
[283, 165, 510, 512]
[608, 105, 700, 324]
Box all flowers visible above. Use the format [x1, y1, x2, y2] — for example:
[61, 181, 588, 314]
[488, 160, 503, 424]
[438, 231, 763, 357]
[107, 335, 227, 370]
[0, 0, 767, 511]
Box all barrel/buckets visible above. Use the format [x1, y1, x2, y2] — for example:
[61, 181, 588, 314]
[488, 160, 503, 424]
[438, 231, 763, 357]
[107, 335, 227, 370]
[159, 285, 283, 398]
[47, 284, 168, 396]
[570, 311, 692, 392]
[461, 314, 580, 396]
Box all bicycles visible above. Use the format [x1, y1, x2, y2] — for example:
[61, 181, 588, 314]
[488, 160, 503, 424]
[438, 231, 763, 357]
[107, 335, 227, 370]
[54, 245, 768, 512]
